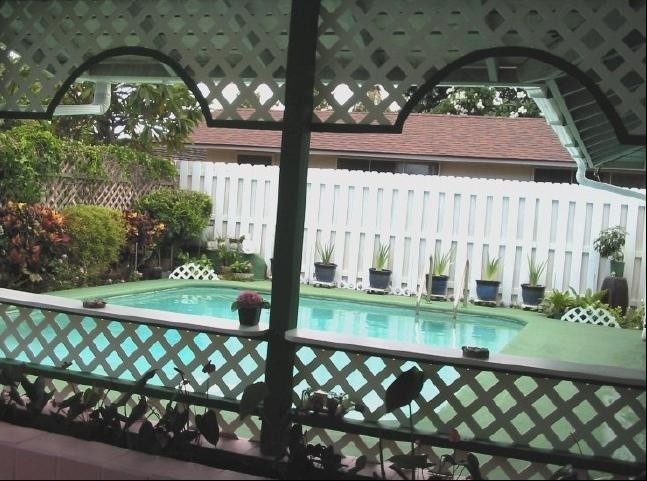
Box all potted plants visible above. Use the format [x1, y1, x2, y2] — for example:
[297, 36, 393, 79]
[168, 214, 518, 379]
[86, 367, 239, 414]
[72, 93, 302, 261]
[367, 241, 394, 290]
[425, 247, 548, 309]
[592, 223, 628, 279]
[312, 241, 337, 284]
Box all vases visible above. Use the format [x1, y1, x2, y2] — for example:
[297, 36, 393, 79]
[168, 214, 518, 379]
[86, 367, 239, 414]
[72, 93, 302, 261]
[237, 310, 262, 326]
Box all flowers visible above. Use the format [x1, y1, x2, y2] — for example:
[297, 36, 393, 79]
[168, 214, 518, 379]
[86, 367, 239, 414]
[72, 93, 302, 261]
[229, 290, 270, 312]
